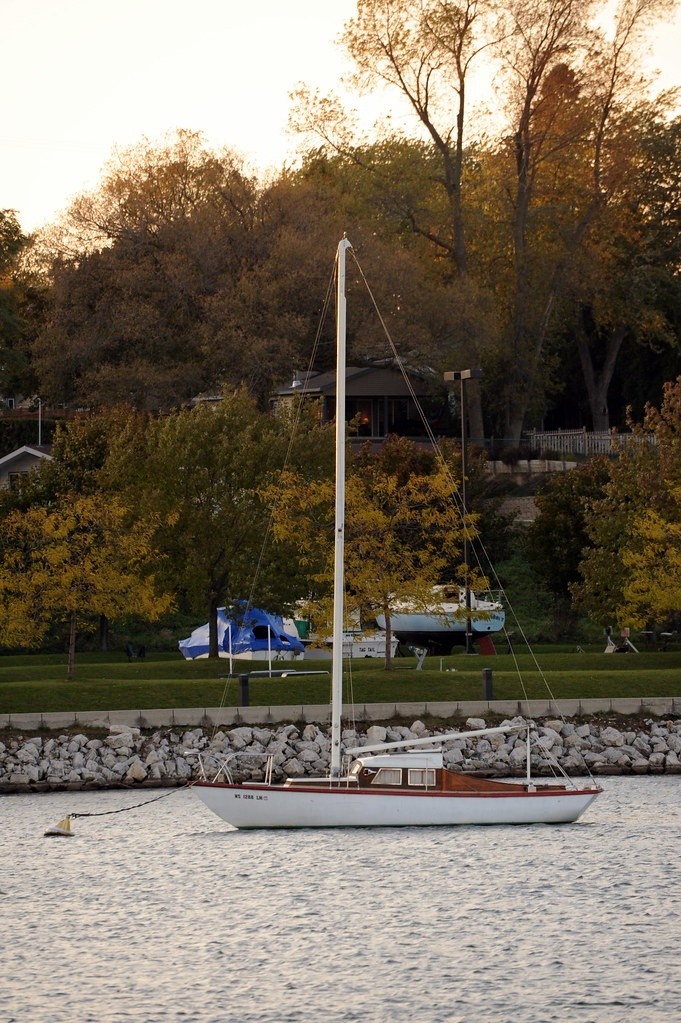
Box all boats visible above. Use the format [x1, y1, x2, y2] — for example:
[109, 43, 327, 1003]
[178, 583, 398, 660]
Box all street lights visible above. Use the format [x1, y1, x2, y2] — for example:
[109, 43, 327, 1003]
[37, 397, 42, 445]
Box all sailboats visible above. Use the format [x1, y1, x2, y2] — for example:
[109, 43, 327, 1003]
[186, 230, 604, 831]
[370, 364, 506, 636]
